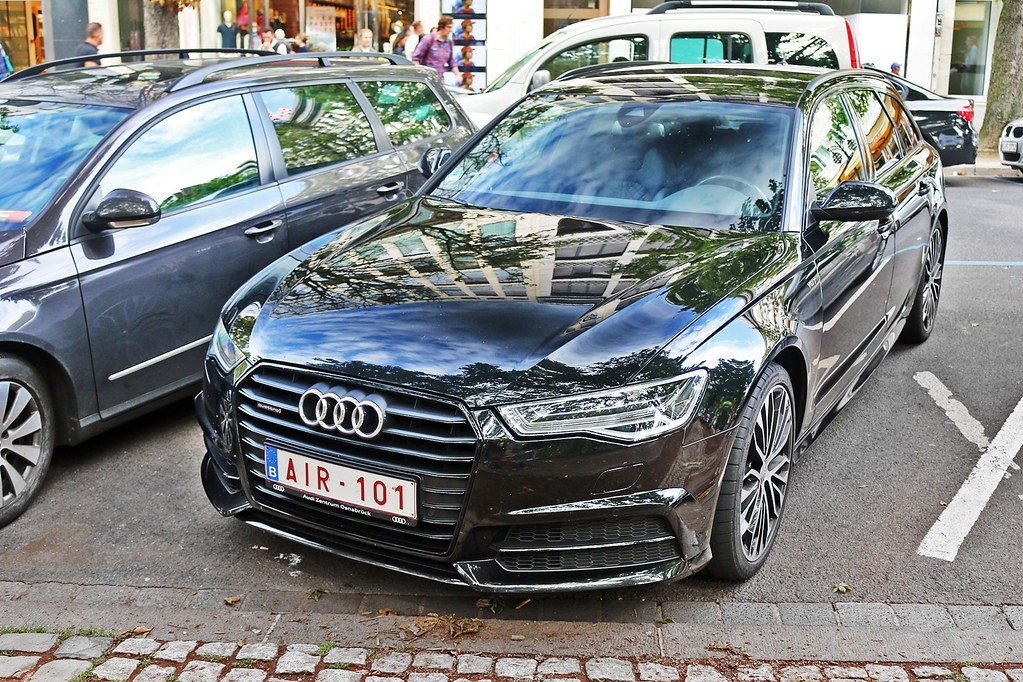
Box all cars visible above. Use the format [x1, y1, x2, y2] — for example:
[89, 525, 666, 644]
[193, 58, 949, 593]
[997, 119, 1023, 172]
[861, 66, 977, 167]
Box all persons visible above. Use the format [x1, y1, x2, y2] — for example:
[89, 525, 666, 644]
[349, 28, 379, 60]
[294, 34, 312, 54]
[216, 11, 241, 58]
[441, 0, 488, 91]
[959, 34, 978, 95]
[890, 62, 904, 76]
[243, 21, 263, 57]
[275, 28, 292, 53]
[258, 29, 287, 57]
[389, 19, 405, 52]
[71, 22, 104, 71]
[391, 19, 463, 91]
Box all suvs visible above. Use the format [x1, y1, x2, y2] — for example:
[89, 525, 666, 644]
[0, 48, 479, 527]
[447, 1, 860, 136]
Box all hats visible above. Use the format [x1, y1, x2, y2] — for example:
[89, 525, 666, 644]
[390, 21, 406, 32]
[462, 72, 475, 79]
[251, 21, 261, 30]
[460, 46, 475, 53]
[890, 62, 903, 70]
[460, 20, 476, 26]
